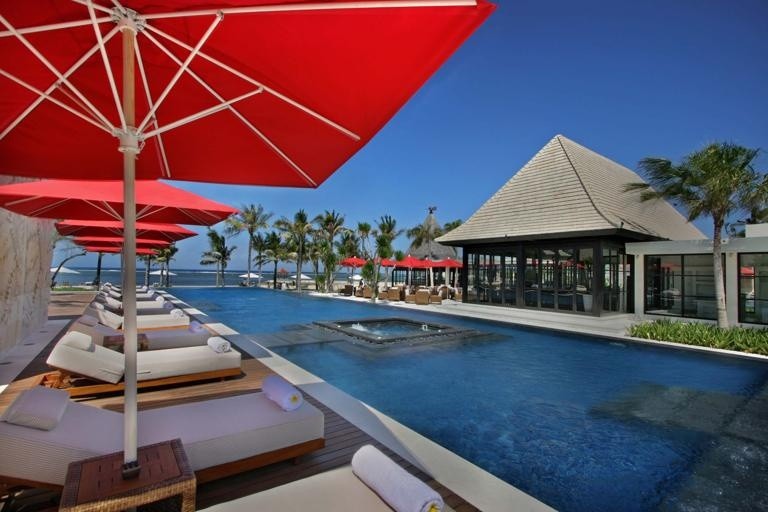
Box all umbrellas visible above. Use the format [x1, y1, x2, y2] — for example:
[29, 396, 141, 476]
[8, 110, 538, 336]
[336, 253, 465, 297]
[0, 1, 500, 465]
[0, 179, 241, 293]
